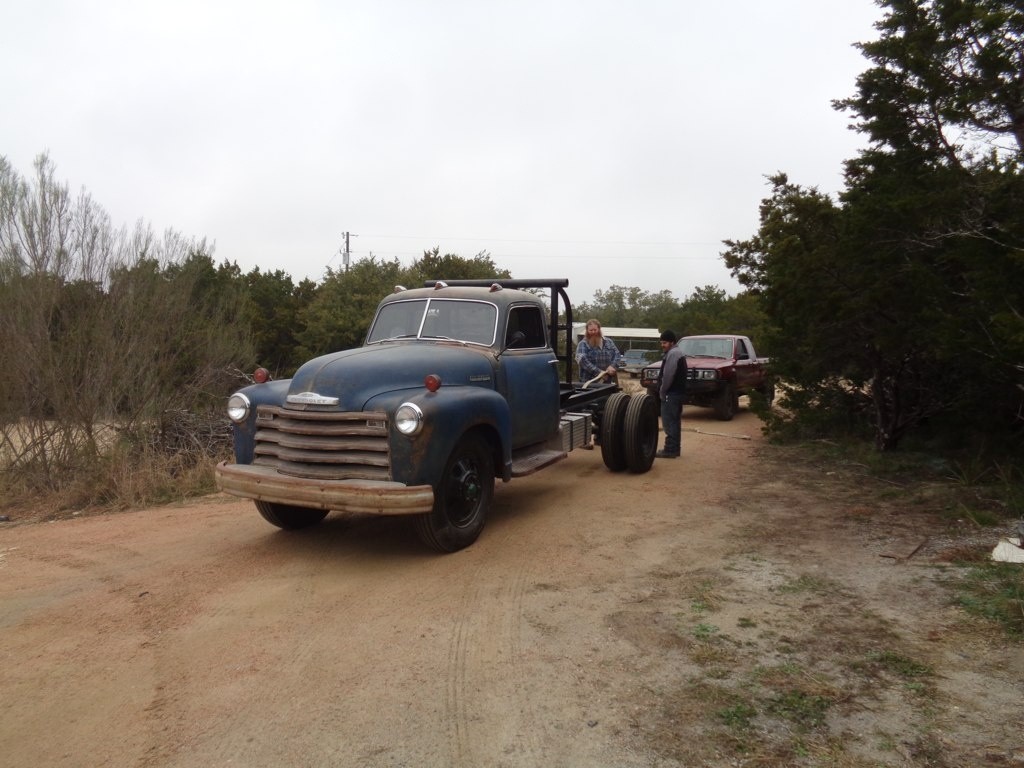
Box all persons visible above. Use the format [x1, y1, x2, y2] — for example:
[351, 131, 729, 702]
[576, 319, 623, 447]
[659, 329, 687, 460]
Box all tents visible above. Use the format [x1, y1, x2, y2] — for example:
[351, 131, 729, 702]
[577, 324, 661, 351]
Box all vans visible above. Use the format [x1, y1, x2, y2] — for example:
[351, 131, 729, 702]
[620, 348, 659, 365]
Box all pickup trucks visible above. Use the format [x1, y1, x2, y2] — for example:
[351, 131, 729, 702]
[641, 334, 781, 421]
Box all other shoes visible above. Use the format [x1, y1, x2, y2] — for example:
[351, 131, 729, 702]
[656, 448, 680, 459]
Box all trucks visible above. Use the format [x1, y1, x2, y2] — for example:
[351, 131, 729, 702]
[214, 274, 660, 554]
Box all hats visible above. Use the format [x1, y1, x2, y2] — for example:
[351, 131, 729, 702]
[660, 330, 676, 342]
